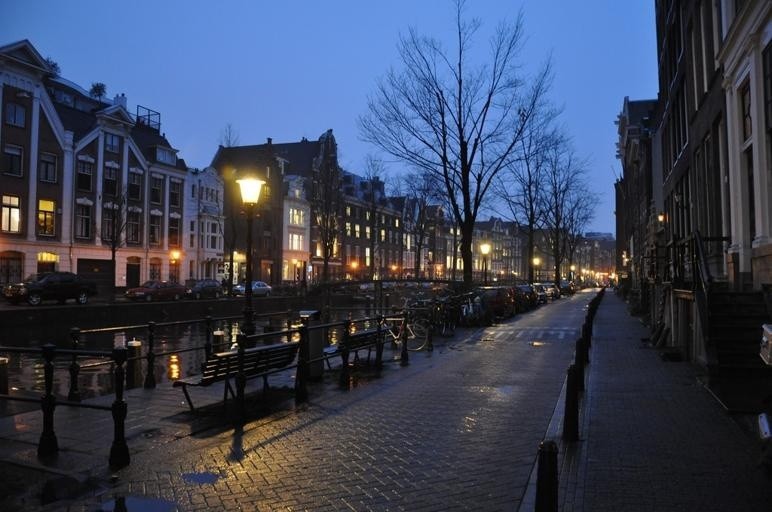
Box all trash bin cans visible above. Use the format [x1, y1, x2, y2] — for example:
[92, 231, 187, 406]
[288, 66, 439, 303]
[299, 311, 324, 380]
[630, 288, 643, 316]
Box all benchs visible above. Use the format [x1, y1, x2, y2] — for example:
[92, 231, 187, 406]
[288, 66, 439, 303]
[323, 325, 399, 368]
[171, 340, 300, 414]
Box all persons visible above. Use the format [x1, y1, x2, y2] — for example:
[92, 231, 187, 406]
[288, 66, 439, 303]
[221, 278, 226, 287]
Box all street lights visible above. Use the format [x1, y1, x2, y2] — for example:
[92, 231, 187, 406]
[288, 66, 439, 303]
[292, 258, 297, 283]
[353, 263, 355, 280]
[172, 251, 180, 283]
[391, 264, 395, 278]
[479, 243, 490, 284]
[582, 269, 585, 283]
[533, 258, 539, 282]
[570, 265, 575, 281]
[233, 173, 268, 434]
[437, 265, 440, 277]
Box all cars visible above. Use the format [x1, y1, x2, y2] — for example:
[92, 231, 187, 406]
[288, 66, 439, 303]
[587, 279, 611, 287]
[233, 281, 271, 297]
[3, 270, 98, 307]
[476, 280, 575, 324]
[124, 281, 187, 302]
[185, 279, 224, 300]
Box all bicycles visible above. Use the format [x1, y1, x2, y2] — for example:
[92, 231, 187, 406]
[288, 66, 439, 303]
[373, 283, 485, 351]
